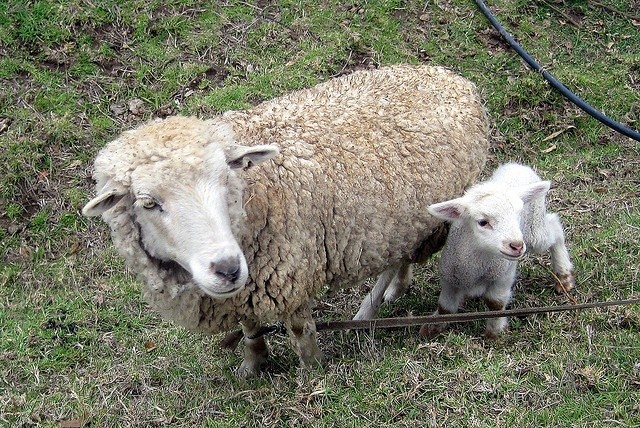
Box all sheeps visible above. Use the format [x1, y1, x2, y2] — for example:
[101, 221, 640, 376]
[418, 161, 575, 340]
[80, 62, 492, 381]
